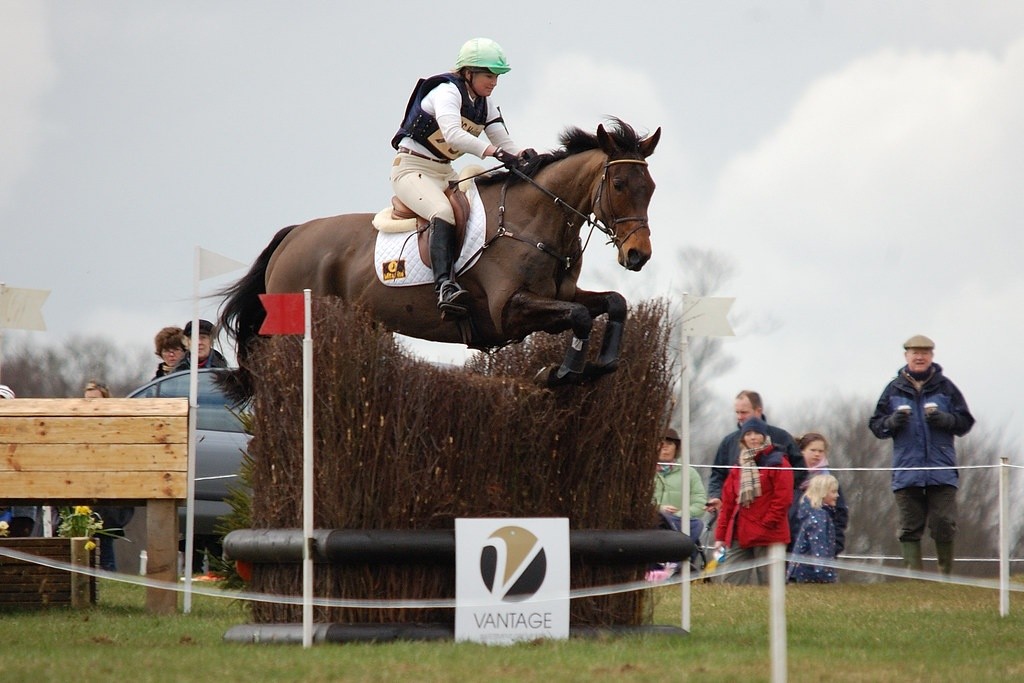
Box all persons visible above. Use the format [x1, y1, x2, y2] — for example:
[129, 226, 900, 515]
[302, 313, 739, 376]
[650, 426, 707, 581]
[868, 335, 975, 579]
[150, 318, 228, 386]
[77, 378, 134, 577]
[390, 38, 537, 309]
[0, 385, 64, 537]
[704, 390, 848, 584]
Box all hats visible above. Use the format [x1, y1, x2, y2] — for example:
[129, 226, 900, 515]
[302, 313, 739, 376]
[183, 319, 218, 338]
[0, 385, 16, 399]
[904, 336, 935, 350]
[658, 429, 681, 449]
[740, 417, 767, 439]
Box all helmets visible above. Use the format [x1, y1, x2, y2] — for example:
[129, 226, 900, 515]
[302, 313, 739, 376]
[455, 38, 512, 76]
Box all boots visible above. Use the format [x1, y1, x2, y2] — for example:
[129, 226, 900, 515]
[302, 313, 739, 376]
[902, 540, 924, 572]
[936, 539, 954, 575]
[430, 217, 469, 321]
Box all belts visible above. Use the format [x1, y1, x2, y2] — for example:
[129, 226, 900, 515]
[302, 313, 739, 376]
[399, 147, 451, 163]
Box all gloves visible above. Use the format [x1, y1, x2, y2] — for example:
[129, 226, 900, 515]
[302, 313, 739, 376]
[924, 410, 955, 427]
[887, 410, 913, 429]
[518, 148, 538, 163]
[493, 146, 520, 171]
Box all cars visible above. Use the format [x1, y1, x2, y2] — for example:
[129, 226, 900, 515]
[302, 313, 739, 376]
[124, 368, 259, 539]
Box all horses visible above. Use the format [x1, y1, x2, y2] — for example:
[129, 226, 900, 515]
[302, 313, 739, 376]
[216, 124, 661, 402]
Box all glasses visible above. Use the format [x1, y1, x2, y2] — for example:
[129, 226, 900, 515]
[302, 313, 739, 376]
[160, 349, 184, 357]
